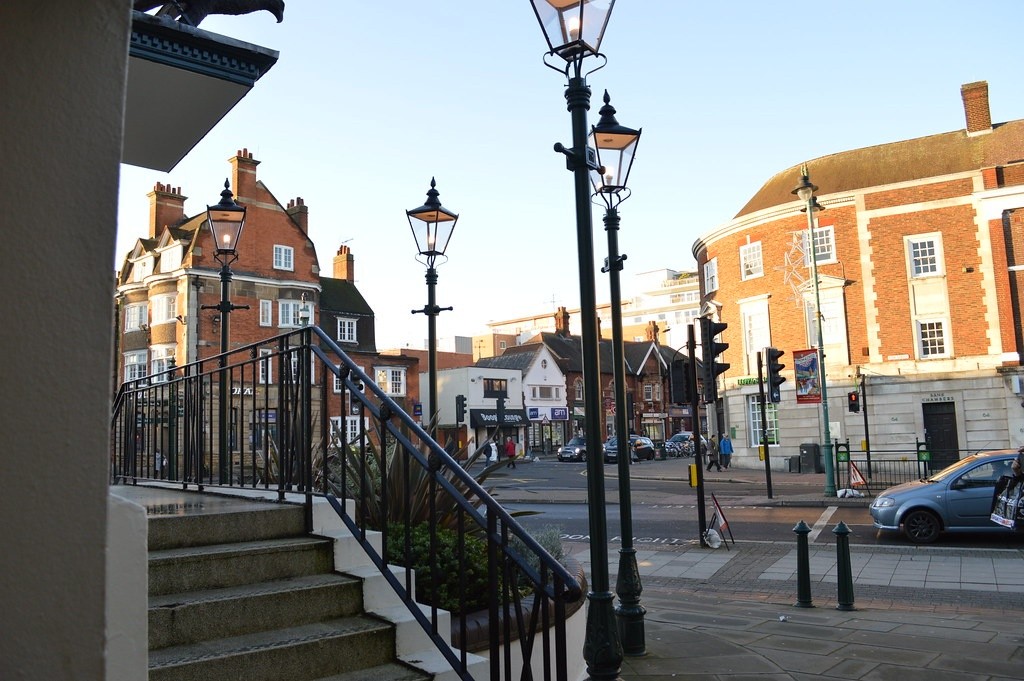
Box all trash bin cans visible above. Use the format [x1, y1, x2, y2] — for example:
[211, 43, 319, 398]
[654, 441, 666, 460]
[800, 444, 820, 474]
[784, 459, 790, 471]
[791, 455, 799, 473]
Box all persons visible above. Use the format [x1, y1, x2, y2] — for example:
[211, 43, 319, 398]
[672, 428, 681, 437]
[483, 437, 498, 468]
[639, 427, 647, 437]
[445, 435, 457, 460]
[156, 448, 161, 478]
[719, 432, 733, 471]
[1012, 445, 1024, 478]
[504, 436, 516, 469]
[630, 427, 636, 434]
[706, 435, 723, 472]
[163, 450, 168, 478]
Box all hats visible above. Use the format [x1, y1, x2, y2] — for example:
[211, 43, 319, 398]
[723, 433, 728, 437]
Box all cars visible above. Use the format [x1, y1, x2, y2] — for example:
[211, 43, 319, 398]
[664, 430, 708, 456]
[557, 436, 606, 462]
[602, 434, 655, 465]
[867, 448, 1024, 546]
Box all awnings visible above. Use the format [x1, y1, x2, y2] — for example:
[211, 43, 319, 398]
[470, 409, 532, 429]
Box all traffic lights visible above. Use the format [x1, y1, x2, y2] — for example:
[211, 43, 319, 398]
[848, 392, 859, 412]
[701, 317, 731, 402]
[765, 347, 787, 403]
[457, 394, 469, 422]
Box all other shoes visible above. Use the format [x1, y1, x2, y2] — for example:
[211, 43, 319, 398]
[718, 470, 722, 472]
[706, 468, 711, 472]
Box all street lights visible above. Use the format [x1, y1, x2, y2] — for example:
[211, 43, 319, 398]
[205, 176, 248, 483]
[790, 174, 838, 498]
[529, 0, 621, 681]
[405, 175, 460, 442]
[657, 328, 673, 442]
[586, 88, 652, 660]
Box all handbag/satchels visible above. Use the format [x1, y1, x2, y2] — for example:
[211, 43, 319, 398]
[707, 449, 714, 456]
